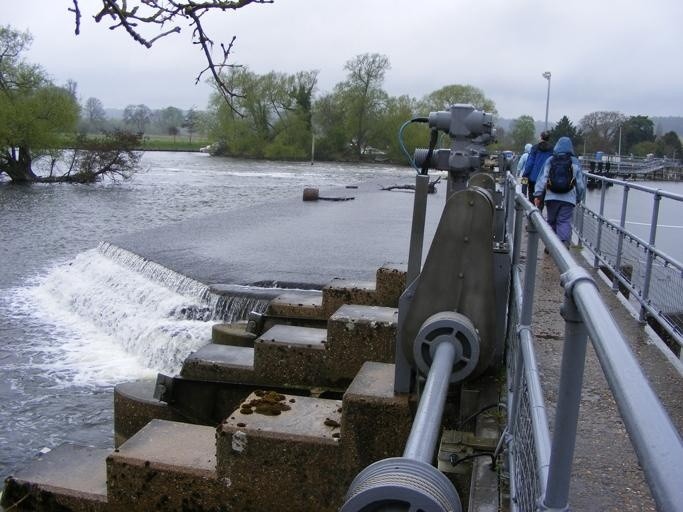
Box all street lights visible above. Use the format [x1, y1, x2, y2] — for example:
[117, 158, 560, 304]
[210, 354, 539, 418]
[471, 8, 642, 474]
[541, 72, 552, 131]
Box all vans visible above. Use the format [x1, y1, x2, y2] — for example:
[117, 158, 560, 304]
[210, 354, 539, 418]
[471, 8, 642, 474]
[502, 151, 513, 161]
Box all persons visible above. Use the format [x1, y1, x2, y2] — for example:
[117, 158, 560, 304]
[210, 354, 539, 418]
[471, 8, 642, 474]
[521, 133, 554, 215]
[516, 142, 536, 197]
[532, 137, 586, 257]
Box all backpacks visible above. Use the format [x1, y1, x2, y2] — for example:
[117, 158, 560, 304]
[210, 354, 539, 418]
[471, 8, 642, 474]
[547, 153, 576, 193]
